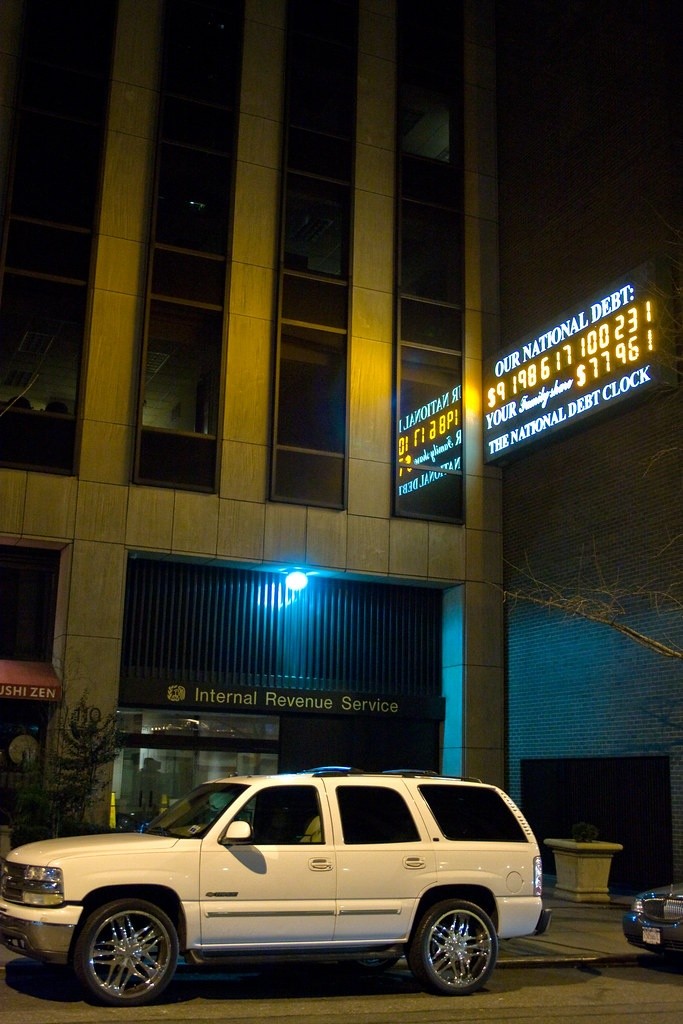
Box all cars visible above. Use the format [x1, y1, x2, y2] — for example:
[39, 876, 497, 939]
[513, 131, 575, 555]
[622, 882, 683, 959]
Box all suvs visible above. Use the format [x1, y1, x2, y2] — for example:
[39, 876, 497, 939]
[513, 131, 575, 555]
[0, 765, 551, 1011]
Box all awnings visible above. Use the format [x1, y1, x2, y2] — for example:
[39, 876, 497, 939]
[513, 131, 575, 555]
[0, 660, 62, 701]
